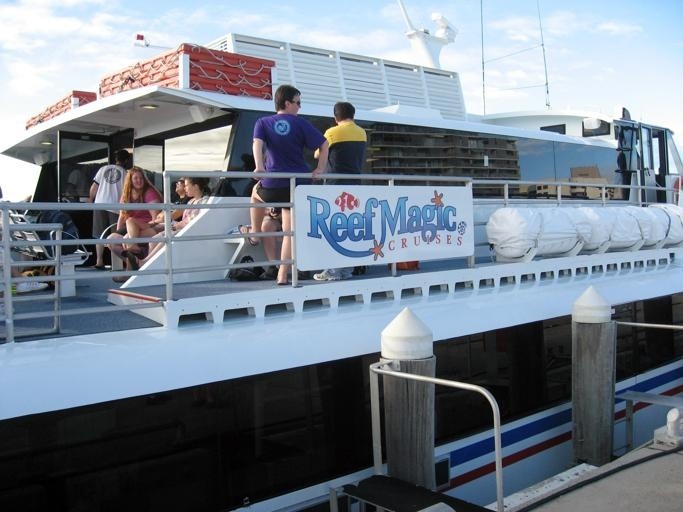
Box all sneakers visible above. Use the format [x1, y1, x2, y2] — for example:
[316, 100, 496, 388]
[313, 268, 352, 281]
[120, 251, 143, 270]
[112, 276, 130, 283]
[16, 281, 48, 293]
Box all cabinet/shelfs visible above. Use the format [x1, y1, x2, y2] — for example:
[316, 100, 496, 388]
[369, 123, 520, 197]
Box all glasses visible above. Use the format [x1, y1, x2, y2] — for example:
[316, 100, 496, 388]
[290, 100, 300, 106]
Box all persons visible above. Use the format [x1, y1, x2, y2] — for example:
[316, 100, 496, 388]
[66, 165, 90, 197]
[259, 206, 310, 280]
[239, 85, 329, 285]
[66, 150, 211, 282]
[313, 102, 367, 280]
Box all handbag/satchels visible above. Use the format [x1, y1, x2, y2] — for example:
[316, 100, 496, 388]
[39, 211, 79, 258]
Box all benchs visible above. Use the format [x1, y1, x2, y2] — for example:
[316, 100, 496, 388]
[0, 200, 92, 298]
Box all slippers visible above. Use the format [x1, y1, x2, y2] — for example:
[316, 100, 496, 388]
[120, 249, 141, 257]
[276, 277, 289, 285]
[238, 225, 257, 248]
[94, 264, 107, 271]
